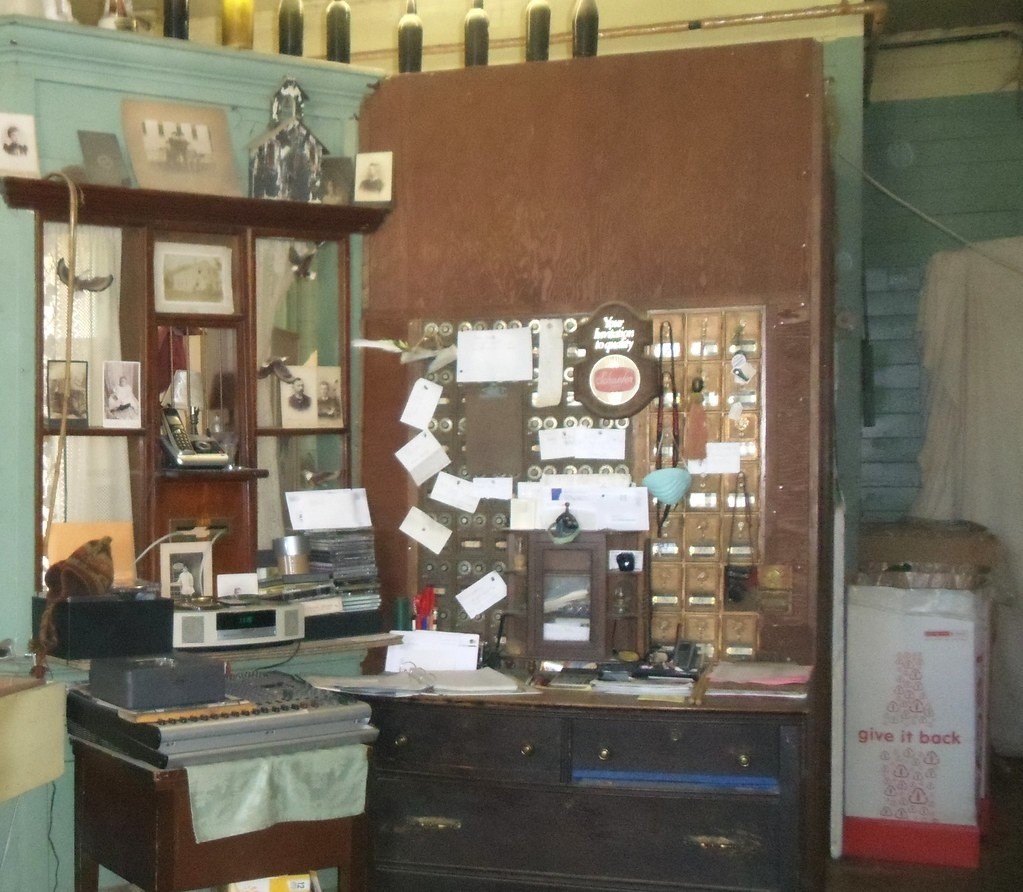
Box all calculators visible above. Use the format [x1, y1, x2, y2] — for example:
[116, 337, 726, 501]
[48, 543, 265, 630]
[548, 666, 600, 687]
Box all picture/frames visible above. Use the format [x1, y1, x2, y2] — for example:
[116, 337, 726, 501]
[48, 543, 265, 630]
[160, 540, 213, 598]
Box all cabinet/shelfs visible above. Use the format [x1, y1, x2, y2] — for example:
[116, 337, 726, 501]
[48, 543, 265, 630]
[3, 175, 403, 670]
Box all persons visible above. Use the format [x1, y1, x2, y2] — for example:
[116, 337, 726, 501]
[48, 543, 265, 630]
[290, 378, 311, 409]
[317, 380, 341, 419]
[110, 377, 133, 417]
[360, 164, 383, 192]
[310, 176, 352, 204]
[179, 564, 195, 599]
[4, 127, 28, 154]
[142, 122, 208, 173]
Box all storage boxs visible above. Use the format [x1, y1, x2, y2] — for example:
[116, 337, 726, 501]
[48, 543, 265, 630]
[32, 590, 174, 659]
[1, 677, 66, 800]
[89, 660, 225, 710]
[844, 586, 991, 869]
[304, 611, 383, 640]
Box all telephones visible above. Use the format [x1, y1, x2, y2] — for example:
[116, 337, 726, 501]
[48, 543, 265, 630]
[157, 403, 230, 468]
[632, 622, 708, 681]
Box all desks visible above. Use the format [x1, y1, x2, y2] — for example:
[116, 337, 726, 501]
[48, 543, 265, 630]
[337, 666, 808, 892]
[70, 741, 372, 891]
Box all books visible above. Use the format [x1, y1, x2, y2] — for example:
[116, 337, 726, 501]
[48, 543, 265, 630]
[298, 663, 544, 696]
[591, 674, 809, 698]
